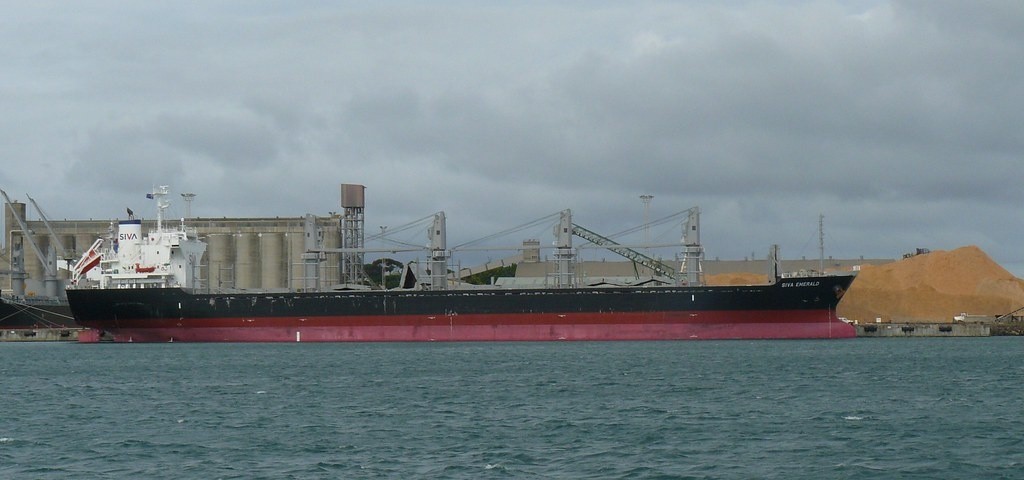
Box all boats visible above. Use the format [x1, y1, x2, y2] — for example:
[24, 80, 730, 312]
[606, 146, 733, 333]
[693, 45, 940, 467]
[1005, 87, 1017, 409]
[56, 185, 860, 344]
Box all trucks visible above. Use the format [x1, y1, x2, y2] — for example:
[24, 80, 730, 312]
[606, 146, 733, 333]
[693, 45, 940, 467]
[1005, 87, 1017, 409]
[953, 313, 989, 325]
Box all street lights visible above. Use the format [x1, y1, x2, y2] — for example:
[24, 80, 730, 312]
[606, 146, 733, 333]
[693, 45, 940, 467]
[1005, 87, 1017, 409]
[179, 191, 197, 226]
[379, 225, 388, 290]
[641, 192, 654, 256]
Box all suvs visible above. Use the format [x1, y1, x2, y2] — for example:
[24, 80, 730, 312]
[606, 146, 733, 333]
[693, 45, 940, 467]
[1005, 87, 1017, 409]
[838, 317, 854, 326]
